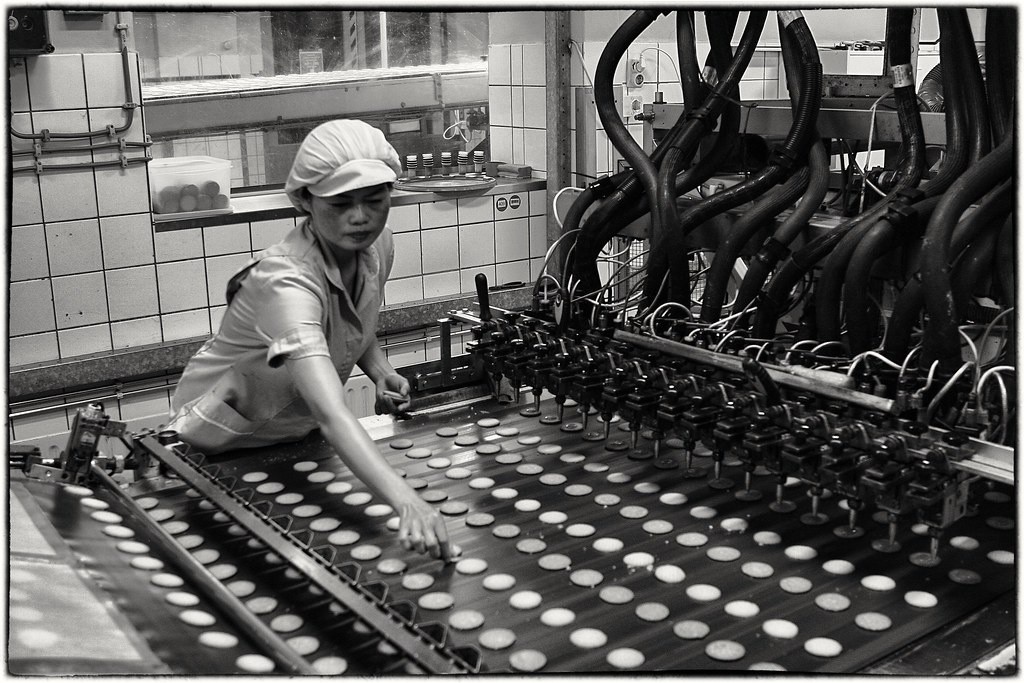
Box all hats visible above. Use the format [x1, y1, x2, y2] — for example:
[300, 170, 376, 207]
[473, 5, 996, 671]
[284, 118, 403, 212]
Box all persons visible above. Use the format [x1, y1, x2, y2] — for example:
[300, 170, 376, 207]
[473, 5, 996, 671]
[171, 116, 455, 563]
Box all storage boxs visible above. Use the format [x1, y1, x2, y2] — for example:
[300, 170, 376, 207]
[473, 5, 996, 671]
[150, 155, 234, 213]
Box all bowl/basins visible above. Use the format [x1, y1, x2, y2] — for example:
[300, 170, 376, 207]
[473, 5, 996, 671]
[485, 161, 506, 177]
